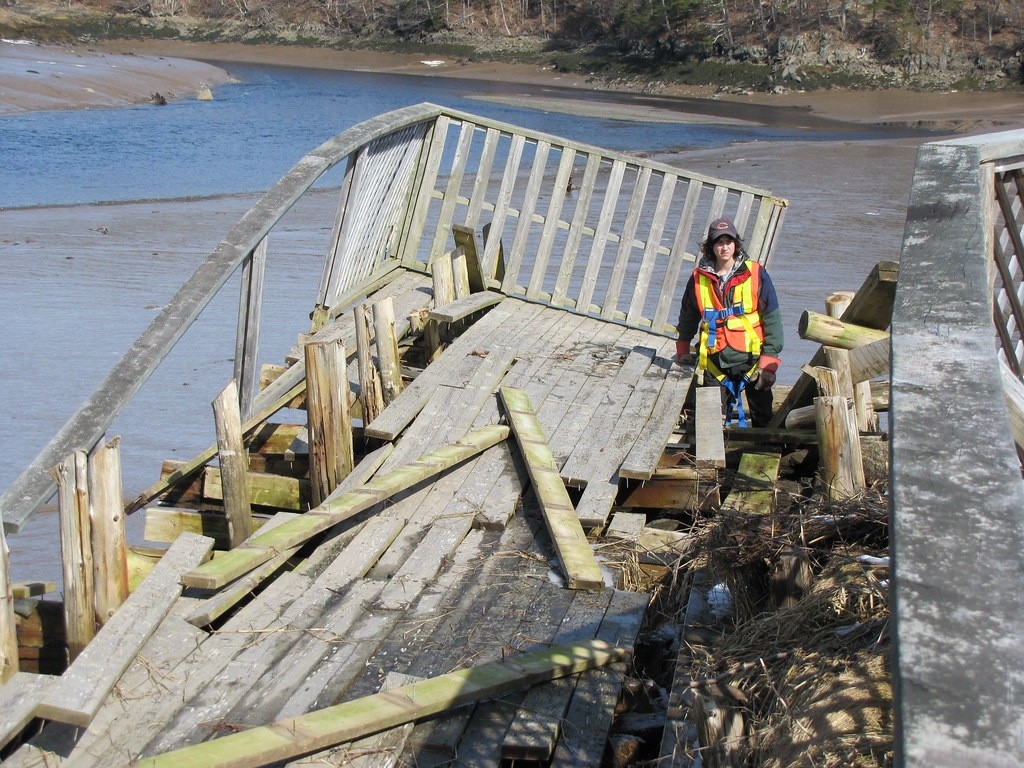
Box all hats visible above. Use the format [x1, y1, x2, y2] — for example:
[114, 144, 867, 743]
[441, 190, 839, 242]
[708, 218, 737, 241]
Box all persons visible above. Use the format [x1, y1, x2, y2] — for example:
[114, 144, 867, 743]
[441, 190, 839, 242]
[676, 219, 784, 434]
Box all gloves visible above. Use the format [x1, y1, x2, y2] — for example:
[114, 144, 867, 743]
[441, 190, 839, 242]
[676, 340, 693, 364]
[750, 354, 781, 392]
